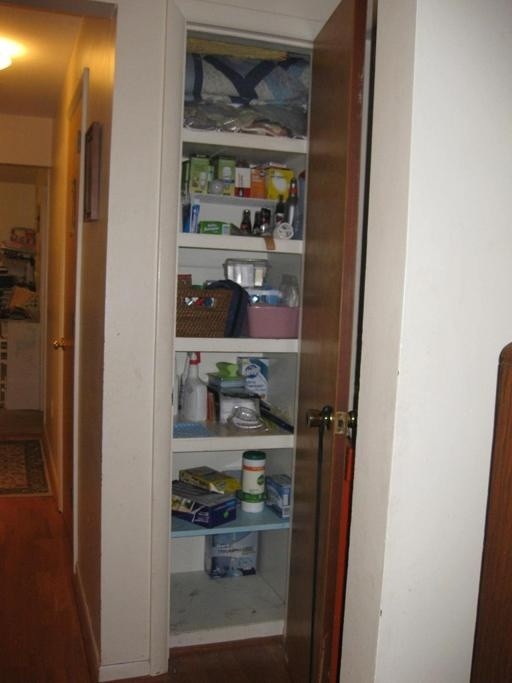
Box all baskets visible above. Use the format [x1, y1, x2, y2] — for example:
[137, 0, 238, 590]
[176, 288, 232, 338]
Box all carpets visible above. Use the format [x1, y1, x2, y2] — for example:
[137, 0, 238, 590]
[0, 436, 53, 498]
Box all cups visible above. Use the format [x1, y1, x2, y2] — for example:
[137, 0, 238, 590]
[241, 450, 266, 514]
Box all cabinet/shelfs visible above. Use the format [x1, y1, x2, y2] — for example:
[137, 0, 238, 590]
[170, 20, 313, 656]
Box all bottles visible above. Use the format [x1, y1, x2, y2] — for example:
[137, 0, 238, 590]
[240, 176, 302, 239]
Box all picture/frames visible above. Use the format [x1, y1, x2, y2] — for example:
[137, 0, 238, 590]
[84, 120, 103, 222]
